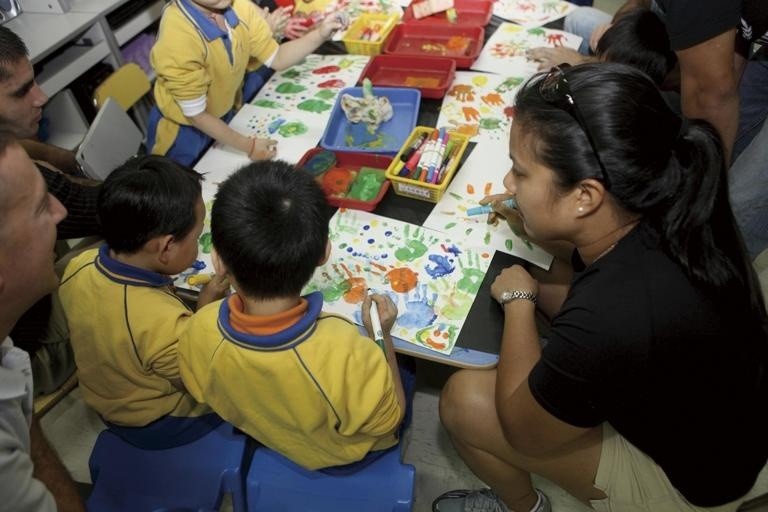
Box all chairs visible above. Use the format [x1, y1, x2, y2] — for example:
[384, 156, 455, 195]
[79, 98, 141, 186]
[92, 62, 153, 120]
[239, 447, 417, 512]
[83, 420, 248, 512]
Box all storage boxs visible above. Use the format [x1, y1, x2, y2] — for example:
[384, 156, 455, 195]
[341, 13, 399, 55]
[321, 85, 422, 159]
[400, 0, 493, 30]
[386, 126, 471, 204]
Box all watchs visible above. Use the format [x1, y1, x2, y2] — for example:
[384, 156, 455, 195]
[496, 291, 538, 309]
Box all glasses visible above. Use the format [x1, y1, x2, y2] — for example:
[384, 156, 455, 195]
[540, 62, 611, 188]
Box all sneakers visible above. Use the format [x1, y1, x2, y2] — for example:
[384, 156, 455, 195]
[431, 487, 552, 512]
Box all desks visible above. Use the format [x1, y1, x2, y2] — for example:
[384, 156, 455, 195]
[63, 0, 170, 134]
[0, 1, 150, 187]
[166, 1, 597, 373]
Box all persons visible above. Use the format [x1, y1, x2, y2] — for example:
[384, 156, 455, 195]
[147, 0, 349, 170]
[524, 0, 682, 122]
[0, 131, 86, 512]
[0, 25, 97, 359]
[652, 1, 763, 268]
[234, 1, 310, 112]
[178, 160, 417, 476]
[431, 62, 764, 512]
[59, 154, 229, 450]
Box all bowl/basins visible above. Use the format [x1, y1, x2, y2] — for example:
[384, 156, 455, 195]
[402, 1, 493, 26]
[320, 87, 420, 151]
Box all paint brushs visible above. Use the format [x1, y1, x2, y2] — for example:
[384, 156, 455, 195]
[466, 198, 523, 217]
[187, 273, 216, 287]
[268, 144, 276, 151]
[366, 287, 388, 362]
[351, 10, 400, 42]
[393, 126, 463, 185]
